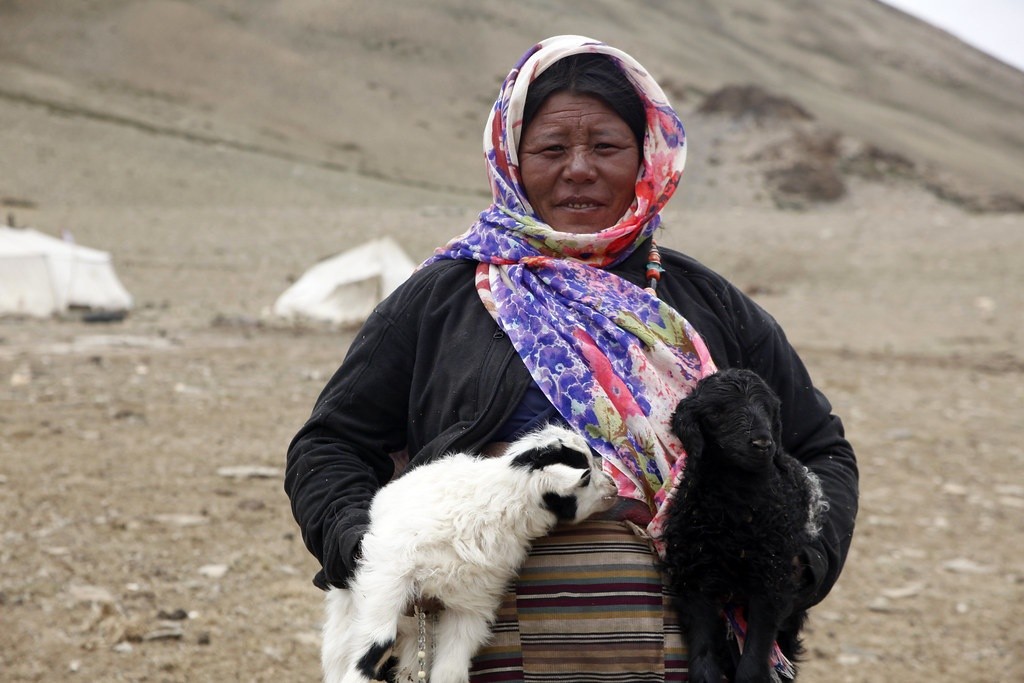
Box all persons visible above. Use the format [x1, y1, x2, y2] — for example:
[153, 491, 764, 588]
[283, 34, 860, 683]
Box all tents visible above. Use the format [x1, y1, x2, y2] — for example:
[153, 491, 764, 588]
[277, 236, 420, 328]
[1, 226, 130, 317]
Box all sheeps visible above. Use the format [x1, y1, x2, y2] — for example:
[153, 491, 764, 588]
[317, 416, 619, 683]
[657, 367, 830, 683]
